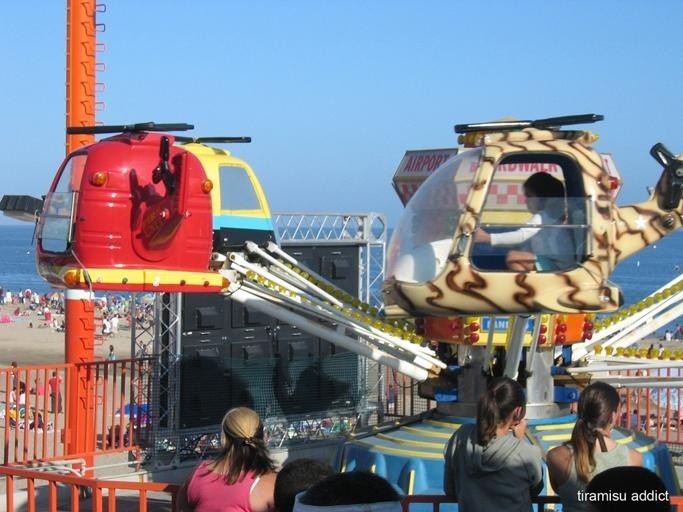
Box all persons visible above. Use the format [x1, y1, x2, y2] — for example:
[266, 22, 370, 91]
[586, 466, 671, 512]
[273, 458, 335, 512]
[176, 407, 278, 512]
[555, 323, 683, 432]
[475, 170, 577, 272]
[442, 376, 545, 512]
[546, 382, 644, 512]
[1, 286, 397, 447]
[291, 467, 406, 512]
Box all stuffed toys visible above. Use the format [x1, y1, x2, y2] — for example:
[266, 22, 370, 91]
[34, 119, 232, 295]
